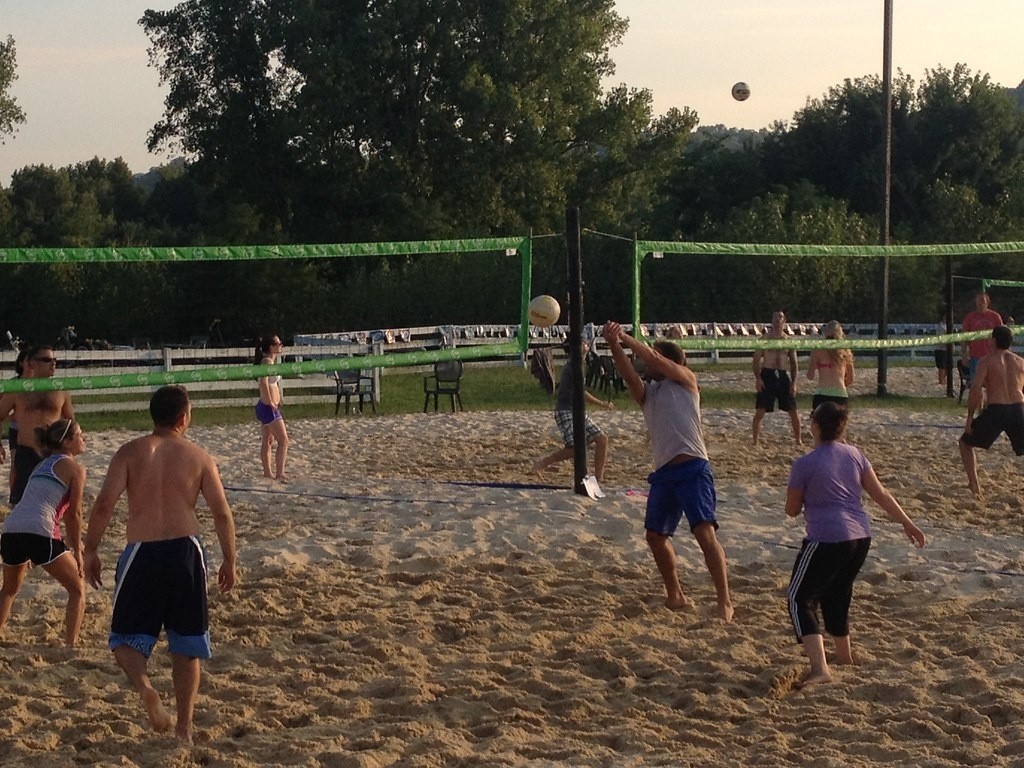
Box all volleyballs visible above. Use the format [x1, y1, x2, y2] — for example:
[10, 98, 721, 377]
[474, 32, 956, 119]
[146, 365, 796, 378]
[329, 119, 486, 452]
[527, 294, 561, 328]
[731, 81, 751, 101]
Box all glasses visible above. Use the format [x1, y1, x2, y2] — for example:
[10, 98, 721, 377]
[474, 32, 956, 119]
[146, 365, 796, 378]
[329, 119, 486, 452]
[809, 410, 814, 419]
[34, 356, 56, 363]
[273, 341, 283, 346]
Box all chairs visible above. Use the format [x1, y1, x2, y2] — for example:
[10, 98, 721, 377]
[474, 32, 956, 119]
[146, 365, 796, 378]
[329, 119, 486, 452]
[333, 368, 376, 417]
[957, 359, 970, 403]
[586, 351, 629, 395]
[424, 359, 464, 412]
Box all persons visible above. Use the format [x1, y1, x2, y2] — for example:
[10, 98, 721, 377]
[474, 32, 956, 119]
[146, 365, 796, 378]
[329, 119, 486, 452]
[608, 321, 737, 625]
[751, 311, 804, 444]
[0, 345, 86, 649]
[534, 336, 613, 485]
[939, 294, 1024, 490]
[254, 334, 289, 484]
[807, 322, 856, 448]
[90, 386, 235, 745]
[783, 400, 927, 689]
